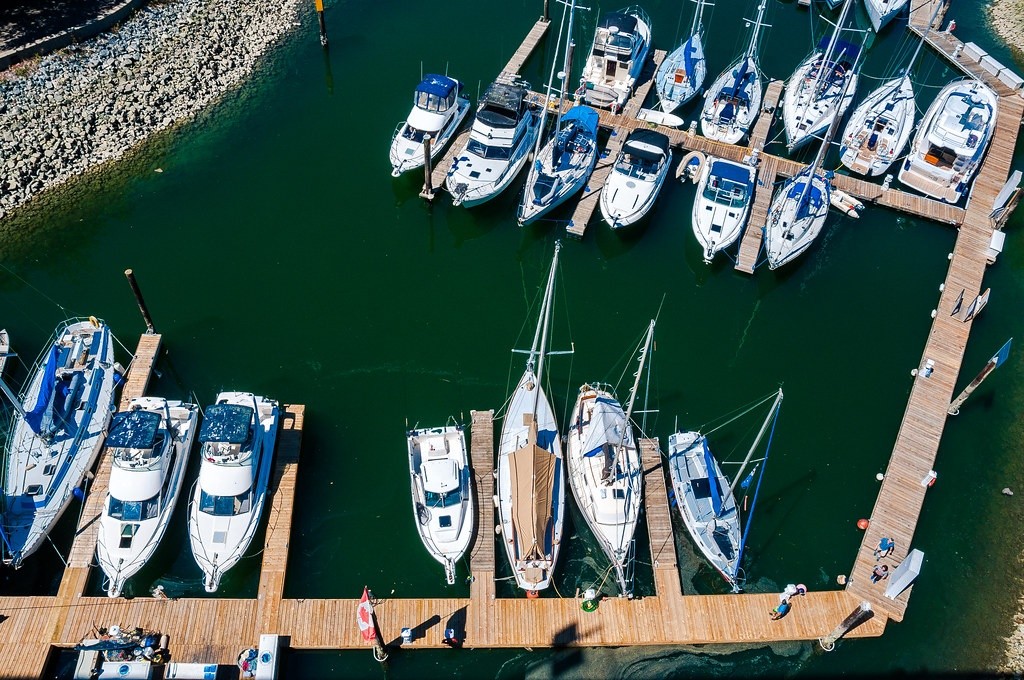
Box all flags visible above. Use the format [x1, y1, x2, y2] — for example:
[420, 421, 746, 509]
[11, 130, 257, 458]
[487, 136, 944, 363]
[356, 588, 377, 642]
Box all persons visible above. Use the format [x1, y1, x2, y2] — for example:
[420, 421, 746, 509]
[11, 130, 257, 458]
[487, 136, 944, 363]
[870, 564, 889, 584]
[873, 537, 895, 561]
[769, 599, 788, 619]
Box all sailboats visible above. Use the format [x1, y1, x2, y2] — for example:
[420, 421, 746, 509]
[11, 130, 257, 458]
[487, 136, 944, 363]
[388, 0, 999, 275]
[0, 237, 786, 680]
